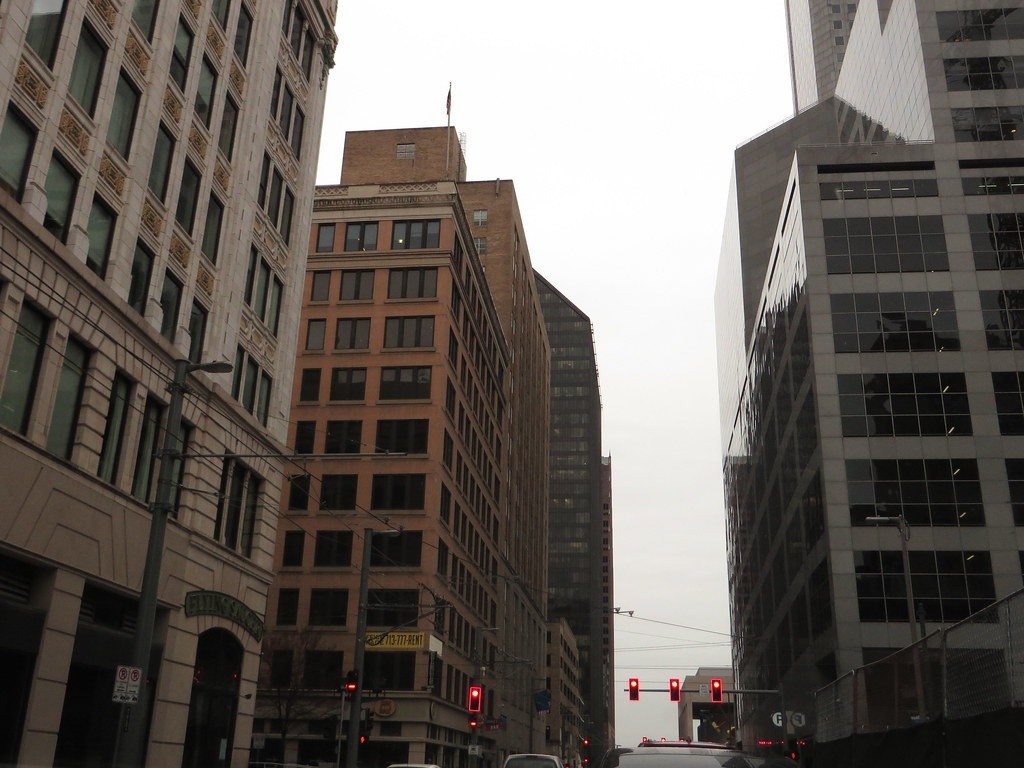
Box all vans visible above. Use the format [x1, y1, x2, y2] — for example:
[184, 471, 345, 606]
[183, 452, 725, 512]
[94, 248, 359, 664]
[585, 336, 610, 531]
[504, 754, 565, 768]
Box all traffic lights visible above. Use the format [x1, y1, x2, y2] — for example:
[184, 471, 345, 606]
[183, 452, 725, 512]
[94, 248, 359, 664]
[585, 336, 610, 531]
[711, 679, 722, 702]
[344, 670, 359, 693]
[629, 679, 638, 700]
[668, 678, 680, 702]
[468, 686, 482, 728]
[642, 737, 685, 742]
[360, 708, 374, 745]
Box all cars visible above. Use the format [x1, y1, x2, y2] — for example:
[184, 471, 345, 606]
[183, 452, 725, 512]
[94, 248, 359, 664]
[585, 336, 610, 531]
[603, 749, 797, 768]
[388, 763, 439, 768]
